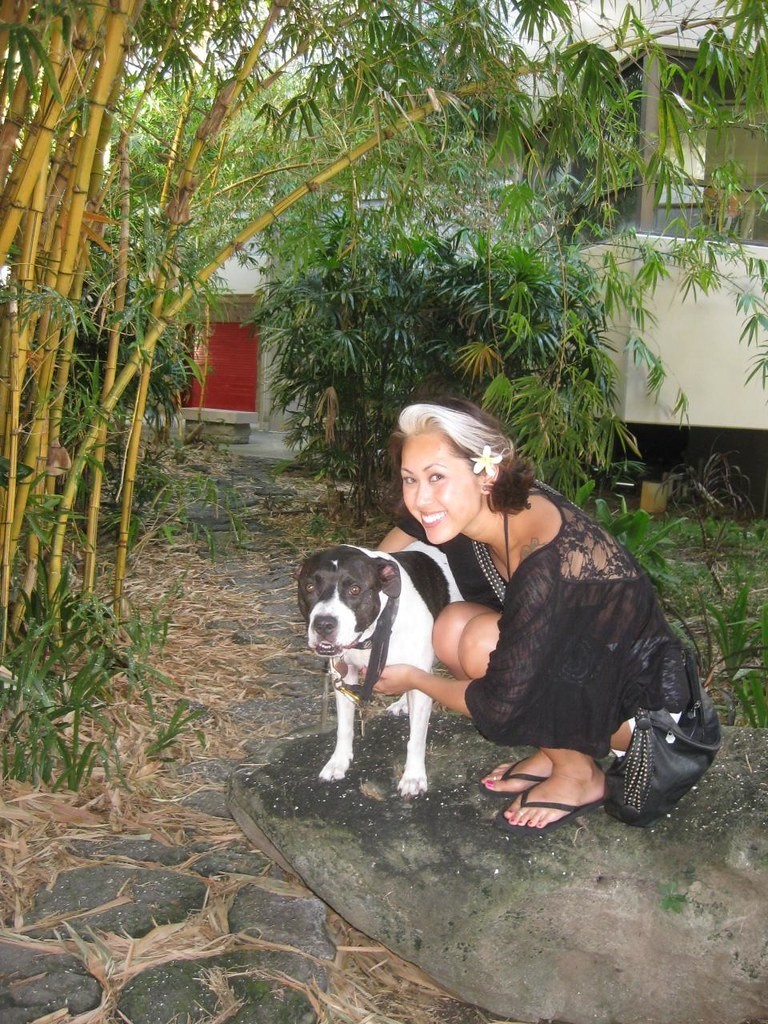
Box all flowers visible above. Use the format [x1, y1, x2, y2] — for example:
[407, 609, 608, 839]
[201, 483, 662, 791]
[470, 445, 503, 476]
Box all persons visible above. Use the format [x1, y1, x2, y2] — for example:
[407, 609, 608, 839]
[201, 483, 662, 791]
[362, 396, 689, 835]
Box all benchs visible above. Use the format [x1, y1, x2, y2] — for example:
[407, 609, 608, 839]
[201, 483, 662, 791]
[181, 406, 257, 443]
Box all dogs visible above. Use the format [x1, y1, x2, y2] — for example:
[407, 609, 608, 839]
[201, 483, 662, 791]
[287, 541, 466, 801]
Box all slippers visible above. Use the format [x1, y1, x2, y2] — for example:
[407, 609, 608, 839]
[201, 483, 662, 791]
[504, 783, 611, 834]
[478, 757, 604, 797]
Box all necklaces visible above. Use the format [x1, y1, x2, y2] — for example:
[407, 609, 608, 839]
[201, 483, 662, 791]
[504, 514, 510, 582]
